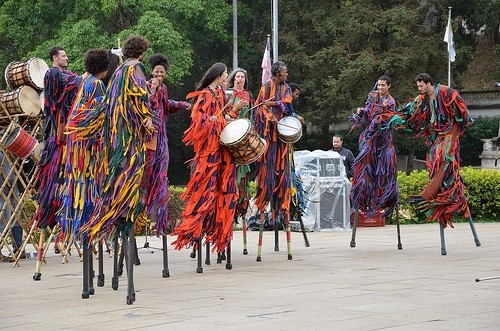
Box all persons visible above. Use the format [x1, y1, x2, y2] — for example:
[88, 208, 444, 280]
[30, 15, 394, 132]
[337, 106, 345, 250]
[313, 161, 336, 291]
[27, 34, 308, 253]
[0, 150, 31, 262]
[330, 134, 355, 181]
[348, 72, 474, 229]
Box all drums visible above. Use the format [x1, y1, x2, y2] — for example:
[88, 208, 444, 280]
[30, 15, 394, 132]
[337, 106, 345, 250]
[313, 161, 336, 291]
[5, 57, 50, 91]
[220, 117, 266, 165]
[40, 91, 44, 112]
[0, 85, 41, 125]
[277, 116, 302, 143]
[4, 127, 38, 160]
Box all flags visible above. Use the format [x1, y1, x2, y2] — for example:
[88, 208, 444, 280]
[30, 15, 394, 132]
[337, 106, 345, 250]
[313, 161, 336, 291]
[261, 37, 273, 84]
[444, 11, 456, 62]
